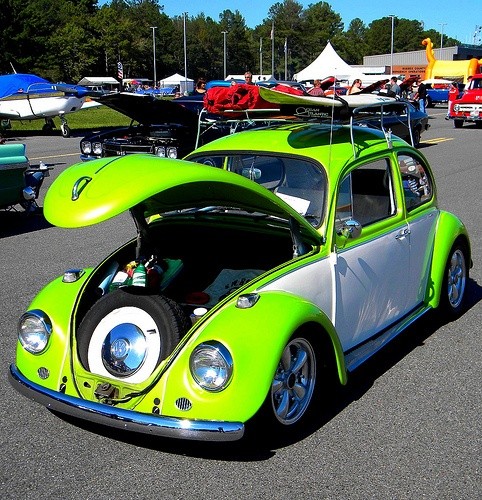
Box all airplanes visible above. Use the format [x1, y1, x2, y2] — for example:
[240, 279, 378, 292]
[0, 62, 102, 138]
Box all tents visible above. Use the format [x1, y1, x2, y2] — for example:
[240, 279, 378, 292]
[294, 42, 363, 90]
[78, 77, 119, 92]
[226, 74, 277, 82]
[160, 73, 193, 92]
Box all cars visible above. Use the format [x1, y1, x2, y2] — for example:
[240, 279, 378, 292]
[416, 77, 482, 129]
[9, 122, 474, 456]
[77, 93, 232, 161]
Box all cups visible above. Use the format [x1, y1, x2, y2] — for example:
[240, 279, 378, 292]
[193, 308, 207, 323]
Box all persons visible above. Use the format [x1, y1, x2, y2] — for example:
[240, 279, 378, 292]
[190, 78, 208, 96]
[345, 79, 363, 95]
[244, 71, 253, 84]
[464, 76, 476, 91]
[230, 78, 236, 87]
[445, 83, 460, 120]
[312, 79, 324, 96]
[413, 79, 427, 112]
[390, 77, 400, 95]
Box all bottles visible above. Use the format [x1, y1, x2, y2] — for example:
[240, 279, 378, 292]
[131, 259, 147, 289]
[108, 264, 132, 294]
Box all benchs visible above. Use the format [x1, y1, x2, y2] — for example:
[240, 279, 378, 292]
[273, 186, 420, 226]
